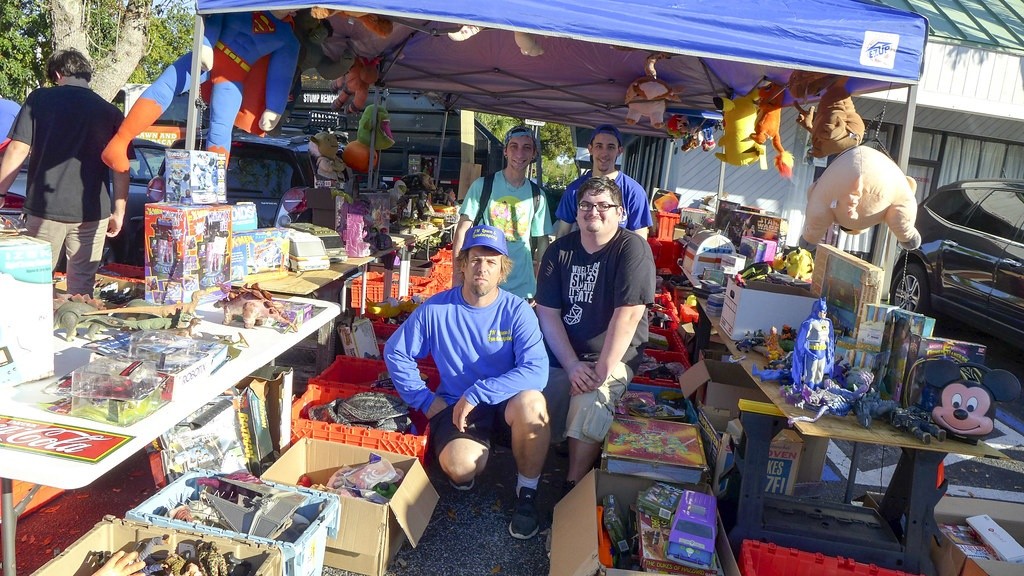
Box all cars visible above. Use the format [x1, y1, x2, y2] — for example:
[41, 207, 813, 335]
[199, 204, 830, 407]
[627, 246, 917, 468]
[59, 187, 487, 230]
[0, 138, 173, 267]
[888, 174, 1024, 357]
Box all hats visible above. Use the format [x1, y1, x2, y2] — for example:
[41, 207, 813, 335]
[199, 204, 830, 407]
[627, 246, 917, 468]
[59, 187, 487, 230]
[459, 225, 508, 258]
[504, 125, 539, 164]
[589, 124, 624, 165]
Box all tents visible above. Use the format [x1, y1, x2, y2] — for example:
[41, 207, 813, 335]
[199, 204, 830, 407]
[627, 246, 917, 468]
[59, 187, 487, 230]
[185, 1, 931, 267]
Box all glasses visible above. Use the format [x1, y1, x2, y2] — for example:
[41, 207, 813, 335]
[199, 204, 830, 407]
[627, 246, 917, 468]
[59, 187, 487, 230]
[577, 202, 620, 212]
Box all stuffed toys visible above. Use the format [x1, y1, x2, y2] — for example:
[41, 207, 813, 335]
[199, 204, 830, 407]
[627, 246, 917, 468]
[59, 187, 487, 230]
[625, 53, 682, 128]
[262, 8, 356, 137]
[712, 89, 765, 167]
[750, 81, 795, 177]
[801, 145, 921, 250]
[447, 24, 544, 56]
[785, 70, 865, 158]
[309, 7, 395, 184]
[663, 112, 717, 154]
[102, 9, 300, 172]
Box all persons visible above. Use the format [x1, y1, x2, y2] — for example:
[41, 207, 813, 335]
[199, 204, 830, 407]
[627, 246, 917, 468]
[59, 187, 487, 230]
[0, 50, 130, 301]
[534, 177, 656, 499]
[451, 125, 554, 310]
[734, 297, 946, 444]
[554, 124, 653, 241]
[384, 225, 551, 539]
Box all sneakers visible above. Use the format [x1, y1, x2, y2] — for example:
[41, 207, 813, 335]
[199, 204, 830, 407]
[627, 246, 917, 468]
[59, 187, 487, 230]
[508, 486, 541, 539]
[546, 481, 575, 521]
[446, 473, 475, 491]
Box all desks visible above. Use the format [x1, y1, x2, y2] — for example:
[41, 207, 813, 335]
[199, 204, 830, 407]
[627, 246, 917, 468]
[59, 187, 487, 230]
[225, 217, 461, 386]
[690, 293, 1010, 560]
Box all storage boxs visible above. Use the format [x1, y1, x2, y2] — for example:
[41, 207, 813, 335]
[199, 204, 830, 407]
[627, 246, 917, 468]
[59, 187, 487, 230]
[551, 200, 1024, 576]
[0, 149, 470, 576]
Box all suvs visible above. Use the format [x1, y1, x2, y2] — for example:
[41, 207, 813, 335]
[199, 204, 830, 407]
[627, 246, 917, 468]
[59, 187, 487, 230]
[148, 128, 349, 227]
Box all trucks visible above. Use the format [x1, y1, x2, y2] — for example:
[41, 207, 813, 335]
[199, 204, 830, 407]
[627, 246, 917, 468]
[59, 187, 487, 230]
[349, 86, 507, 197]
